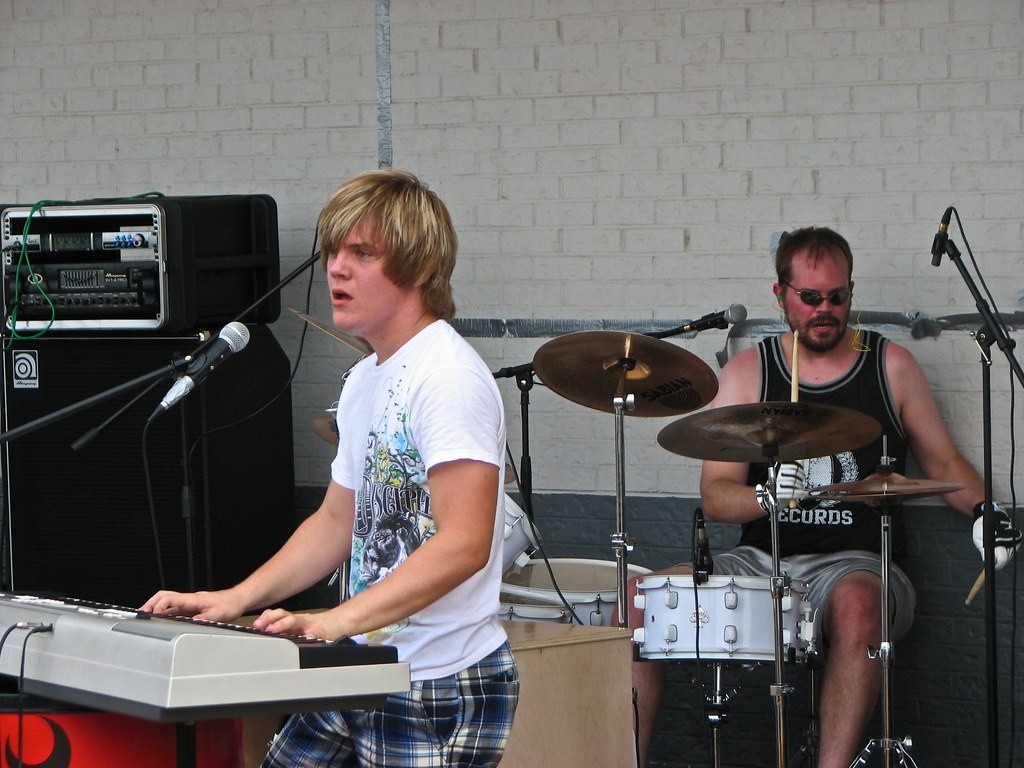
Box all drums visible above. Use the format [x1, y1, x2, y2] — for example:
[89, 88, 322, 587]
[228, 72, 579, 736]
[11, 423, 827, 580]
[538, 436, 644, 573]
[499, 555, 656, 627]
[503, 495, 545, 581]
[632, 572, 822, 663]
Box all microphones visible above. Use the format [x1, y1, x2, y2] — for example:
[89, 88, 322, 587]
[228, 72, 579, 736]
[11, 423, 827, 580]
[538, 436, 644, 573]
[931, 206, 953, 268]
[694, 507, 713, 575]
[147, 321, 251, 422]
[679, 303, 748, 334]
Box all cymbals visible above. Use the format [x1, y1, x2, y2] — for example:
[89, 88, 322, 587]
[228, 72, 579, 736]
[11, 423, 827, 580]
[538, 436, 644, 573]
[285, 306, 373, 356]
[805, 470, 967, 503]
[658, 403, 881, 462]
[529, 329, 722, 419]
[311, 412, 515, 485]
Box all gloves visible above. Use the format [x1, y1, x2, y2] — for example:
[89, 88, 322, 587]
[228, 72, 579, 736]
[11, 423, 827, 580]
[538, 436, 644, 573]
[756, 459, 805, 521]
[972, 501, 1023, 570]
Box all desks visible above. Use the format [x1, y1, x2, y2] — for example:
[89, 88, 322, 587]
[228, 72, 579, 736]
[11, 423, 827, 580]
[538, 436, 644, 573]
[231, 608, 638, 768]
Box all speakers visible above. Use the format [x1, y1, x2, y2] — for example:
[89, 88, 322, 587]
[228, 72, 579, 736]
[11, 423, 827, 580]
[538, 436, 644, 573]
[0, 319, 297, 616]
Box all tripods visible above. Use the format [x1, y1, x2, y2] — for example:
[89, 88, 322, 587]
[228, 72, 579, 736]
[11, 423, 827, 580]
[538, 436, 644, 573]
[846, 502, 921, 768]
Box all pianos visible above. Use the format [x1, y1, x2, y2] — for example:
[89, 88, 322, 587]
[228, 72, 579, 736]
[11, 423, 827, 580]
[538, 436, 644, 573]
[0, 584, 421, 730]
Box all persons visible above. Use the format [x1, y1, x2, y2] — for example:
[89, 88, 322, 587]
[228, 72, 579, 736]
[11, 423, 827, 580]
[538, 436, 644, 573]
[137, 169, 520, 768]
[610, 225, 1021, 768]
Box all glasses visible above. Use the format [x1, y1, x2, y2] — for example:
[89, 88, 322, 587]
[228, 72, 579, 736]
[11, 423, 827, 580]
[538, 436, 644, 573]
[784, 281, 849, 305]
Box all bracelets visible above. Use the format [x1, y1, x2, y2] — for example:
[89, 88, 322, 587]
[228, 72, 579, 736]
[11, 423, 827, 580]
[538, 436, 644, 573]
[755, 484, 770, 513]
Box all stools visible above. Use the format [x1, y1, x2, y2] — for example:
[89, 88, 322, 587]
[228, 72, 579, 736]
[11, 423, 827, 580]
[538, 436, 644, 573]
[786, 640, 824, 768]
